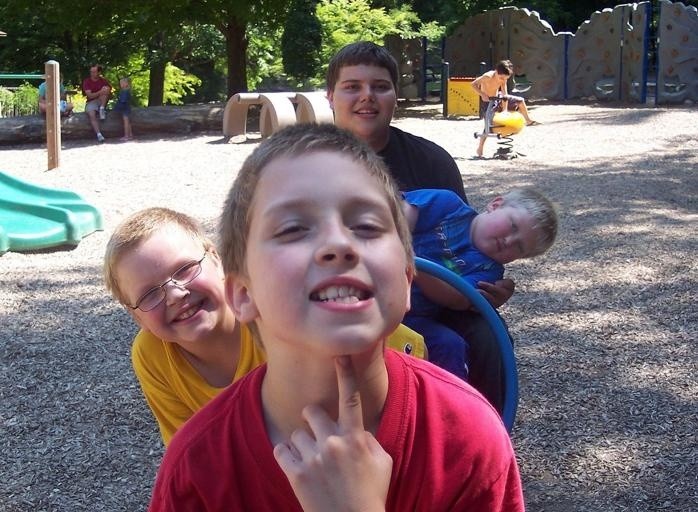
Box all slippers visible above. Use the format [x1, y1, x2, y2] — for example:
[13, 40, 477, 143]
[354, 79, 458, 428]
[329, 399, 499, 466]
[473, 155, 484, 158]
[525, 120, 542, 126]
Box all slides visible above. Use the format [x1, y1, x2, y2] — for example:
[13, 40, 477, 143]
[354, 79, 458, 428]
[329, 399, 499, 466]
[0, 172, 103, 253]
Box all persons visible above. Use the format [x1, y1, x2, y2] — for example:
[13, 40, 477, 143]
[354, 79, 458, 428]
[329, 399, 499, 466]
[104, 205, 431, 452]
[82, 63, 114, 143]
[323, 41, 516, 313]
[113, 78, 135, 142]
[469, 56, 545, 159]
[144, 120, 525, 511]
[37, 73, 74, 118]
[393, 185, 559, 381]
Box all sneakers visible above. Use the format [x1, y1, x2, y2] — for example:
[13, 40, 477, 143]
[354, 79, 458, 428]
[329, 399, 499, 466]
[97, 132, 104, 141]
[120, 136, 128, 140]
[128, 136, 132, 139]
[99, 109, 105, 119]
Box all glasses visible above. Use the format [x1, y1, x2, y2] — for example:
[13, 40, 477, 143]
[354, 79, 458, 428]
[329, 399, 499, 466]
[124, 249, 207, 312]
